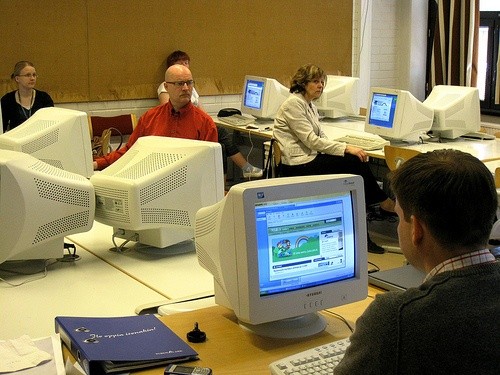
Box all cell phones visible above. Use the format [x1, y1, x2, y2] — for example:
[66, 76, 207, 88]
[164, 365, 213, 375]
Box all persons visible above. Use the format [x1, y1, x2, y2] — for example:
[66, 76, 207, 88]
[94, 64, 219, 170]
[0, 60, 54, 132]
[271, 63, 398, 254]
[333, 149, 500, 375]
[157, 50, 266, 178]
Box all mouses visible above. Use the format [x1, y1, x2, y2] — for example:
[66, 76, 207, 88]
[247, 124, 259, 129]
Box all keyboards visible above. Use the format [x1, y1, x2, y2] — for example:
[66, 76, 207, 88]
[217, 114, 256, 126]
[269, 337, 351, 375]
[462, 131, 496, 140]
[334, 133, 390, 151]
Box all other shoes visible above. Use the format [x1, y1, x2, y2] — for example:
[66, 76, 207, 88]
[367, 241, 385, 253]
[379, 207, 399, 217]
[243, 166, 263, 180]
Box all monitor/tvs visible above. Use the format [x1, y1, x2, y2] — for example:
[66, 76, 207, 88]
[364, 87, 435, 146]
[422, 85, 481, 139]
[0, 107, 226, 277]
[193, 173, 369, 338]
[242, 75, 291, 123]
[313, 75, 361, 118]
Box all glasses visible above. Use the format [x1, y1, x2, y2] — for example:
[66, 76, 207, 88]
[166, 80, 195, 87]
[18, 73, 38, 77]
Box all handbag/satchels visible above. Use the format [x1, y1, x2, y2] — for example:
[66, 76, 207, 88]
[92, 127, 124, 158]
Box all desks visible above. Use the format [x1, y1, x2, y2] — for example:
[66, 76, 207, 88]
[0, 115, 500, 375]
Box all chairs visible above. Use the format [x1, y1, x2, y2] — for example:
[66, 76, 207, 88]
[269, 134, 282, 178]
[89, 115, 137, 138]
[381, 144, 423, 171]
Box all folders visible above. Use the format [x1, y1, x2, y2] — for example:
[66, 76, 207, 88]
[55, 314, 200, 375]
